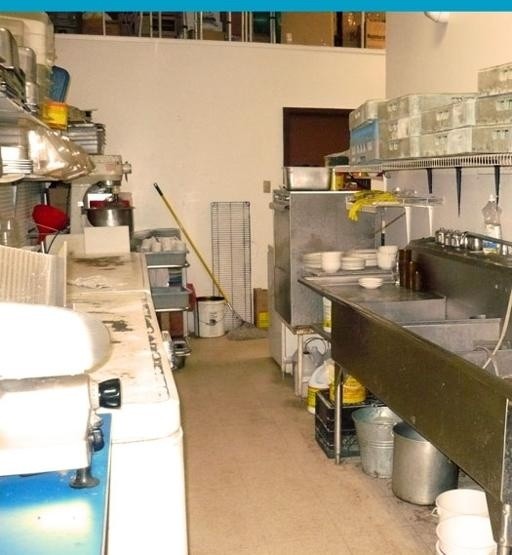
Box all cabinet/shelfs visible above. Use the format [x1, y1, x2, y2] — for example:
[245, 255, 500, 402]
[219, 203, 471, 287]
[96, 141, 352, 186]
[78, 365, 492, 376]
[0, 93, 70, 184]
[139, 234, 195, 373]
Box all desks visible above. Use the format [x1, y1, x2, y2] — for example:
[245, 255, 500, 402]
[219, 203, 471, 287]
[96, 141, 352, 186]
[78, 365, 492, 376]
[276, 315, 332, 395]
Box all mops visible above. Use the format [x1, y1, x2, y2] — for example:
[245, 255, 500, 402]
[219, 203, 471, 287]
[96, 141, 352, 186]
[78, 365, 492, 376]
[154, 182, 268, 341]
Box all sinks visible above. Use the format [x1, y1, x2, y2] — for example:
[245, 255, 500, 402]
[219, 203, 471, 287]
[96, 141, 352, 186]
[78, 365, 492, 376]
[401, 320, 512, 355]
[461, 349, 512, 380]
[354, 297, 501, 323]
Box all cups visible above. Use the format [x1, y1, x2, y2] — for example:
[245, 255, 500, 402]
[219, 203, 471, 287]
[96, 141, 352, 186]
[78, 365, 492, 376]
[141, 237, 186, 252]
[397, 249, 412, 288]
[321, 251, 343, 273]
[0, 217, 19, 247]
[434, 227, 483, 251]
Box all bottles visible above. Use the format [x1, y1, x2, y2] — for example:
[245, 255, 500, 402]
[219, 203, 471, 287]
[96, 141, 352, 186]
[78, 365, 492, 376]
[413, 269, 423, 290]
[408, 260, 416, 290]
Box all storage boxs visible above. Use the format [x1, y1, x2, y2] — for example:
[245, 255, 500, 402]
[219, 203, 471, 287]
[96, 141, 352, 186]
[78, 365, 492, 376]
[73, 11, 389, 48]
[312, 386, 384, 460]
[252, 287, 268, 330]
[347, 63, 512, 168]
[281, 164, 334, 191]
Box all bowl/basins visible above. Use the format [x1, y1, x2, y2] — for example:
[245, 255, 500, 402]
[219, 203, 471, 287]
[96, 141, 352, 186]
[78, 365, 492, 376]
[376, 245, 398, 269]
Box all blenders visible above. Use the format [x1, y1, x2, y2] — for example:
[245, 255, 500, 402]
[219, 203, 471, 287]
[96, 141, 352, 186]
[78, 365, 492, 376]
[68, 153, 134, 236]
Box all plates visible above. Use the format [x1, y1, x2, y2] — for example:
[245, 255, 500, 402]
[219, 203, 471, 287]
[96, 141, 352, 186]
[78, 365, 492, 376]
[339, 257, 366, 270]
[303, 252, 322, 269]
[358, 278, 383, 290]
[353, 247, 378, 267]
[2, 159, 34, 175]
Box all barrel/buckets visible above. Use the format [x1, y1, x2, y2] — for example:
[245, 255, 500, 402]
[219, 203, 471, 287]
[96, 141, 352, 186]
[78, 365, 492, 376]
[429, 488, 490, 522]
[305, 358, 336, 415]
[390, 421, 459, 507]
[196, 296, 225, 338]
[434, 515, 498, 555]
[351, 406, 402, 480]
